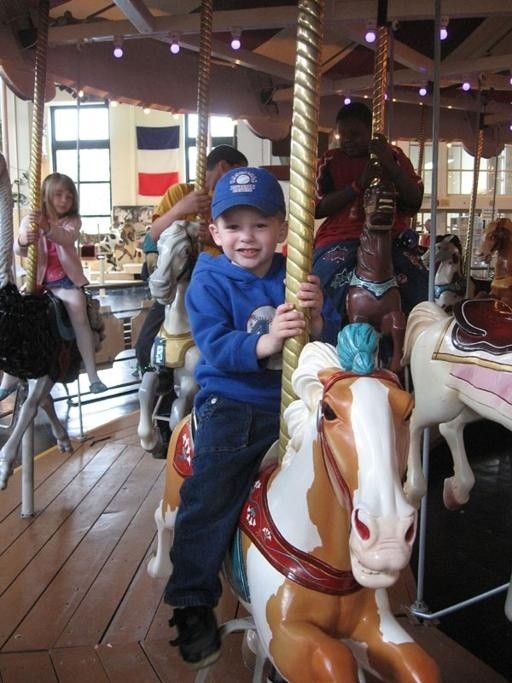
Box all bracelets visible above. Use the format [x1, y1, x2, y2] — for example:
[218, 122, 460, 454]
[351, 178, 362, 194]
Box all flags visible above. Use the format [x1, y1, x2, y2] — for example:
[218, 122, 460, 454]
[136, 125, 180, 196]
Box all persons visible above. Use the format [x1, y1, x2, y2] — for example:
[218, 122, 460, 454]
[311, 102, 430, 345]
[135, 144, 248, 376]
[164, 165, 344, 669]
[420, 219, 432, 246]
[0, 173, 108, 404]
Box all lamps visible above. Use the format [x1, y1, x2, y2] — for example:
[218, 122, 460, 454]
[340, 76, 473, 111]
[165, 30, 184, 54]
[108, 32, 126, 59]
[435, 14, 452, 41]
[360, 15, 382, 44]
[223, 25, 248, 52]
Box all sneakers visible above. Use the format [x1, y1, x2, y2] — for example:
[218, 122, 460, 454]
[0, 382, 18, 400]
[170, 604, 222, 671]
[90, 382, 108, 394]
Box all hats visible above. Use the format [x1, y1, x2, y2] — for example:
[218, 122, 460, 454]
[211, 167, 284, 220]
